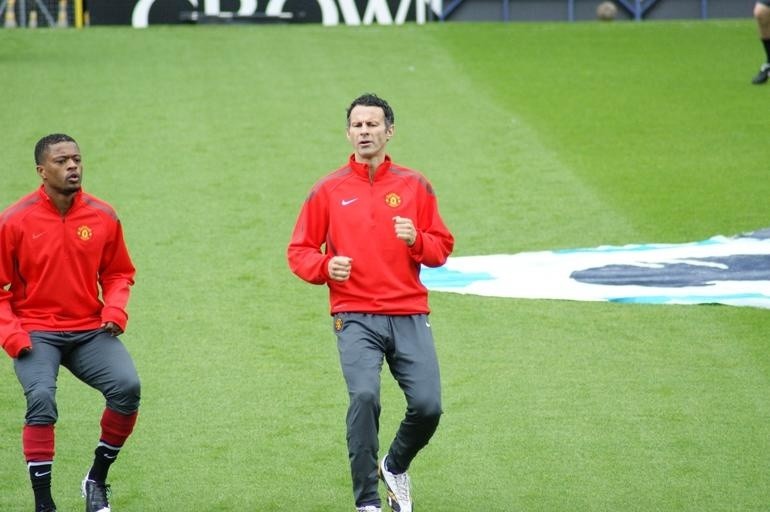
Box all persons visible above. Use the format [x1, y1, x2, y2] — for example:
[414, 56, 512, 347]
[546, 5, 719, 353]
[0, 133, 142, 511]
[751, 0, 769, 85]
[287, 96, 454, 512]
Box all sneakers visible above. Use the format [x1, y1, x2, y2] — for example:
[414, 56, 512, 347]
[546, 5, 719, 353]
[752, 64, 770, 84]
[82, 471, 111, 512]
[356, 454, 414, 512]
